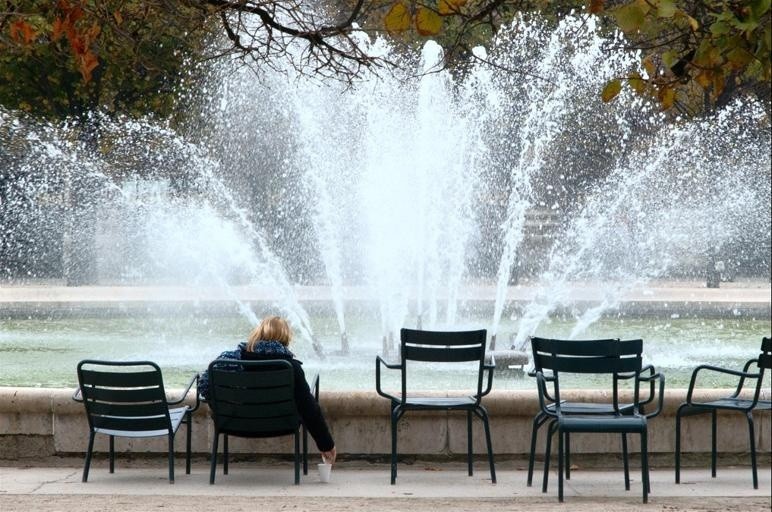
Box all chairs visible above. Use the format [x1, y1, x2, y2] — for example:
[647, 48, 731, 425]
[527, 337, 665, 505]
[200, 360, 320, 484]
[375, 328, 497, 484]
[675, 336, 772, 489]
[73, 359, 199, 484]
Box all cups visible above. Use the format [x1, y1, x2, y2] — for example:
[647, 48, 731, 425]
[317, 463, 332, 483]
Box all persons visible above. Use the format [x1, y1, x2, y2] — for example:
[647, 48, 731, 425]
[196, 314, 337, 465]
